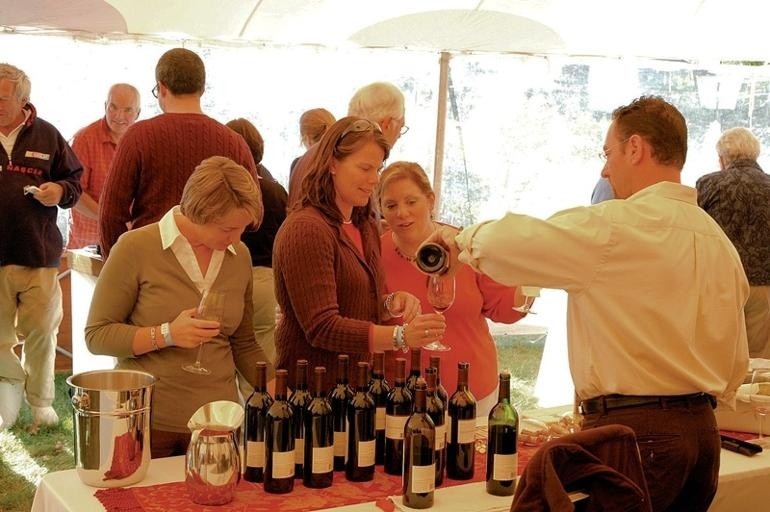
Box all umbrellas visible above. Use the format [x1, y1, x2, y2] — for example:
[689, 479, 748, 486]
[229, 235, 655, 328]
[0, 2, 132, 42]
[351, 3, 561, 220]
[106, 0, 279, 47]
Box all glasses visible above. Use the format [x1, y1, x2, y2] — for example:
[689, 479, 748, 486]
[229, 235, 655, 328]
[596, 136, 630, 161]
[151, 83, 163, 97]
[389, 116, 410, 135]
[337, 120, 383, 139]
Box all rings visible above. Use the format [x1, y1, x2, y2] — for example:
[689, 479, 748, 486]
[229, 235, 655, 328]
[424, 329, 429, 337]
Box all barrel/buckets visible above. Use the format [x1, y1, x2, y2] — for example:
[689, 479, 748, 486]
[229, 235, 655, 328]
[66, 368, 161, 490]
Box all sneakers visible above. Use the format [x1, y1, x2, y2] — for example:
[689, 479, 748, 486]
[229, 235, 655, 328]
[29, 404, 62, 429]
[1, 380, 24, 430]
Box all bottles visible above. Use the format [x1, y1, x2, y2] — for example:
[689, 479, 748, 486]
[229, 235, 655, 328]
[486, 373, 519, 496]
[385, 359, 412, 477]
[423, 366, 444, 487]
[304, 367, 334, 487]
[290, 359, 314, 478]
[416, 242, 446, 276]
[346, 361, 376, 482]
[264, 369, 294, 493]
[429, 355, 451, 418]
[446, 363, 476, 481]
[405, 347, 428, 404]
[243, 363, 273, 481]
[403, 378, 435, 508]
[329, 354, 355, 471]
[368, 350, 389, 465]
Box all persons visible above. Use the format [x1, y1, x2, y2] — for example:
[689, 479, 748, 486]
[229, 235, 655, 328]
[81, 154, 275, 461]
[0, 62, 84, 434]
[375, 158, 543, 420]
[65, 82, 142, 278]
[346, 80, 410, 154]
[223, 116, 289, 400]
[692, 124, 770, 368]
[289, 106, 336, 182]
[94, 46, 263, 268]
[269, 115, 448, 407]
[412, 93, 756, 512]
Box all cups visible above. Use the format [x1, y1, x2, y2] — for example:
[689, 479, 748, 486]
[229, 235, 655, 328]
[518, 417, 560, 446]
[512, 285, 538, 316]
[423, 273, 456, 352]
[749, 370, 770, 446]
[181, 289, 227, 376]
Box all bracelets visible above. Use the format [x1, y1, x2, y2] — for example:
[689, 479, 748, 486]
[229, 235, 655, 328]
[147, 325, 161, 350]
[390, 322, 408, 355]
[382, 291, 404, 320]
[159, 322, 173, 348]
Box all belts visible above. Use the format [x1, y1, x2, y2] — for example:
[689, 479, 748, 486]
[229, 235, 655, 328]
[578, 393, 706, 414]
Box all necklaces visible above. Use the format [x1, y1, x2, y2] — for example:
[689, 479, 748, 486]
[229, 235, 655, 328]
[392, 222, 438, 266]
